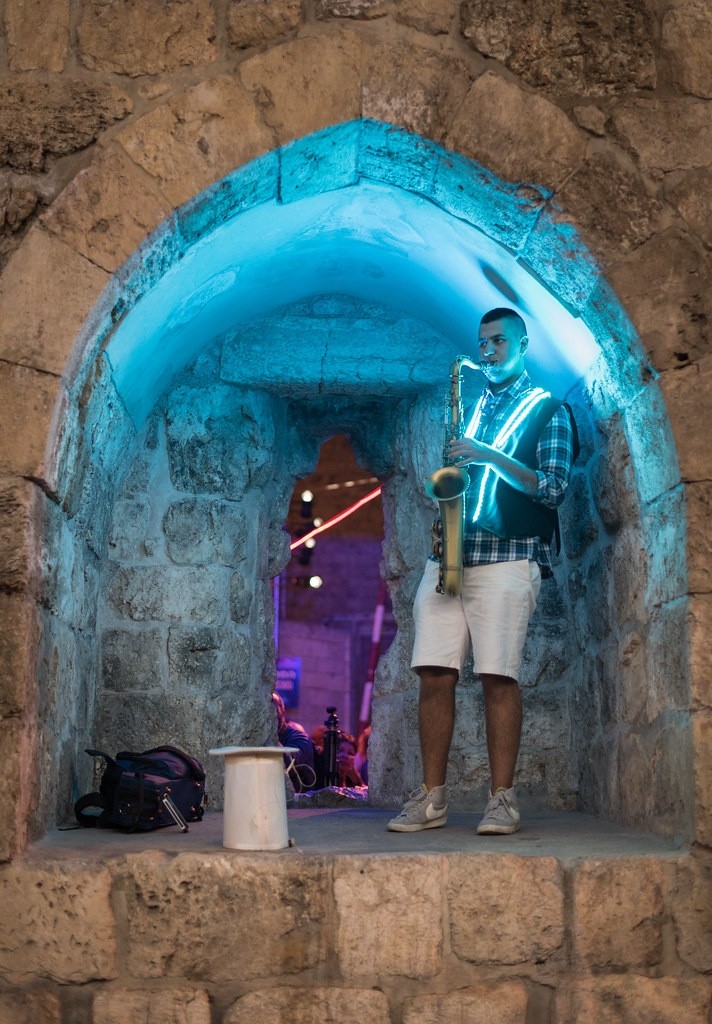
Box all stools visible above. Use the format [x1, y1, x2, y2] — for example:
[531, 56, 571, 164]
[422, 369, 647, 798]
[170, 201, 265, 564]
[208, 745, 300, 851]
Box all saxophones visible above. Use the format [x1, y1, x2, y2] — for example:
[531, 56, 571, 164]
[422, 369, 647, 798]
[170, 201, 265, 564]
[426, 354, 498, 596]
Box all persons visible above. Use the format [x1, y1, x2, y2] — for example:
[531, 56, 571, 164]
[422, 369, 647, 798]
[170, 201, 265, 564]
[386, 307, 576, 838]
[270, 691, 371, 790]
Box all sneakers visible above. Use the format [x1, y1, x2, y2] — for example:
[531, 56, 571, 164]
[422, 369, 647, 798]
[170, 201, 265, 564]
[476, 786, 520, 836]
[386, 782, 448, 831]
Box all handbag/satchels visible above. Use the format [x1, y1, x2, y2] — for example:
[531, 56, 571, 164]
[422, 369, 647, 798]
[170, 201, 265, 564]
[72, 743, 207, 831]
